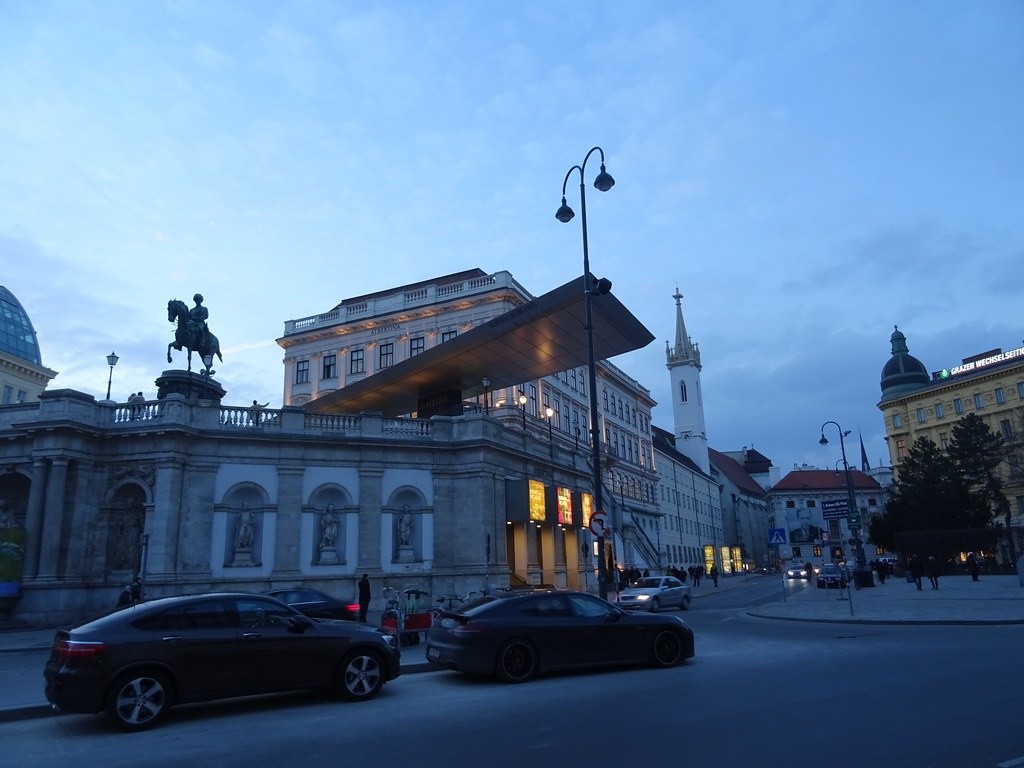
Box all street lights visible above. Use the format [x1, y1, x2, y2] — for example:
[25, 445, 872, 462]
[554, 146, 615, 602]
[835, 459, 858, 511]
[483, 375, 491, 415]
[519, 396, 527, 429]
[107, 351, 119, 400]
[819, 421, 864, 571]
[547, 408, 553, 443]
[573, 420, 579, 450]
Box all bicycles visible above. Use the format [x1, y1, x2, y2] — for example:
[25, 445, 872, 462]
[381, 587, 514, 651]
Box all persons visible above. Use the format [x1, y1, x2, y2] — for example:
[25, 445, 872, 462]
[249, 400, 270, 426]
[117, 503, 145, 571]
[129, 391, 145, 420]
[398, 504, 415, 544]
[967, 554, 980, 581]
[321, 503, 339, 546]
[117, 583, 139, 606]
[665, 564, 704, 588]
[790, 509, 824, 547]
[358, 573, 371, 622]
[838, 559, 847, 566]
[710, 563, 718, 587]
[190, 294, 208, 347]
[730, 557, 735, 576]
[877, 559, 890, 584]
[910, 552, 939, 591]
[805, 562, 812, 583]
[618, 566, 650, 588]
[234, 502, 255, 547]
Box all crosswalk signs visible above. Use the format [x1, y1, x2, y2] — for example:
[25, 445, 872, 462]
[770, 530, 785, 544]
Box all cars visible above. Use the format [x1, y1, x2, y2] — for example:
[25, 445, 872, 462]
[878, 558, 898, 564]
[817, 561, 856, 588]
[264, 588, 359, 621]
[426, 589, 696, 685]
[613, 576, 691, 613]
[43, 592, 402, 731]
[788, 564, 808, 579]
